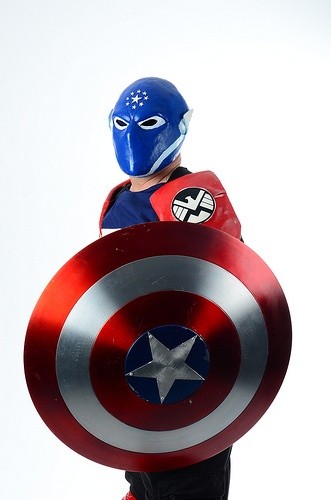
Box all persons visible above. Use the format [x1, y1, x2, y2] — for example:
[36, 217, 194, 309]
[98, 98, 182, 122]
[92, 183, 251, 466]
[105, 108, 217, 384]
[89, 77, 246, 500]
